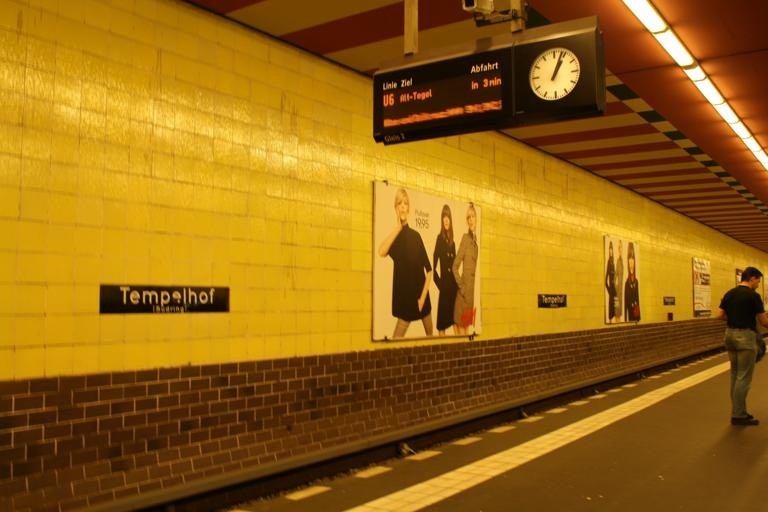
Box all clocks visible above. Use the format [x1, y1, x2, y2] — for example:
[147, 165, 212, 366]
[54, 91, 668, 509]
[529, 48, 580, 101]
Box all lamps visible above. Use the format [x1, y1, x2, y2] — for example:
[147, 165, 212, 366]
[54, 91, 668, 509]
[622, 0, 768, 171]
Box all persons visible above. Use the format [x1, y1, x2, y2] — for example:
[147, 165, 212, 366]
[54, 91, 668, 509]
[378, 188, 433, 339]
[433, 204, 459, 336]
[605, 241, 617, 320]
[614, 239, 626, 321]
[451, 201, 478, 336]
[714, 267, 768, 425]
[624, 242, 640, 321]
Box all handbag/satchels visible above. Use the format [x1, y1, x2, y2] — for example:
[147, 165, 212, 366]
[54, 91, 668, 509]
[757, 333, 765, 362]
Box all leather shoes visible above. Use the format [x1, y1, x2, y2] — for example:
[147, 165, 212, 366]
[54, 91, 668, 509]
[732, 414, 760, 425]
